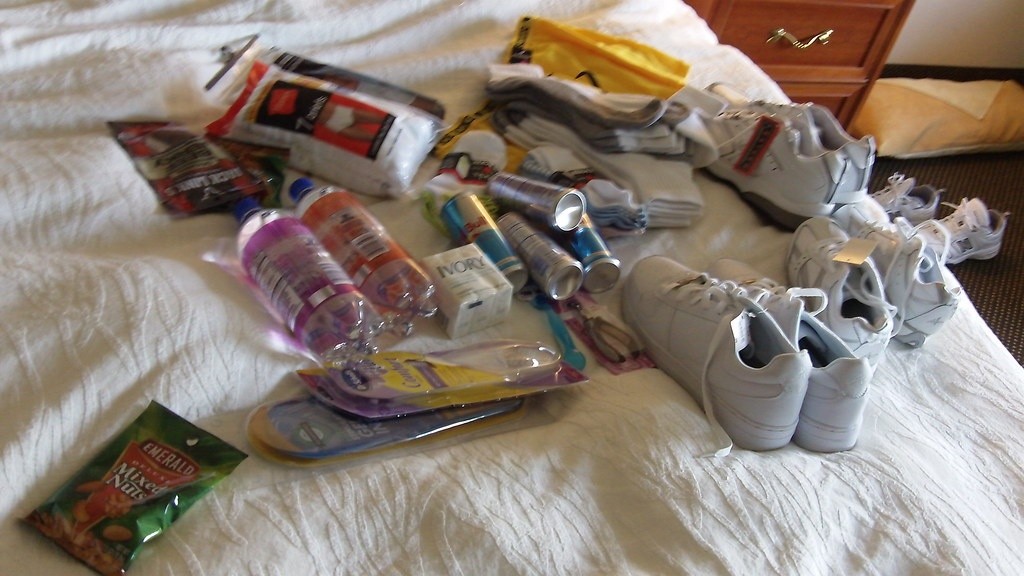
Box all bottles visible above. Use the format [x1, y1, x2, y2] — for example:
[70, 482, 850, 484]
[228, 197, 381, 369]
[283, 174, 436, 340]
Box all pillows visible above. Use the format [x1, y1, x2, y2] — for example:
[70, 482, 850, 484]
[852, 78, 1024, 159]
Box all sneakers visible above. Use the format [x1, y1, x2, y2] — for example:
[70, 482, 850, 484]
[697, 110, 851, 231]
[621, 255, 812, 452]
[830, 203, 961, 348]
[710, 258, 872, 453]
[786, 217, 899, 357]
[871, 171, 945, 226]
[703, 80, 875, 216]
[918, 196, 1011, 265]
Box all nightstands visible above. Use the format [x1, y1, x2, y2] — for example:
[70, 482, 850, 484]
[683, 0, 916, 135]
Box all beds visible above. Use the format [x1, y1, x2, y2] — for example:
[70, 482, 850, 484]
[0, 0, 1024, 575]
